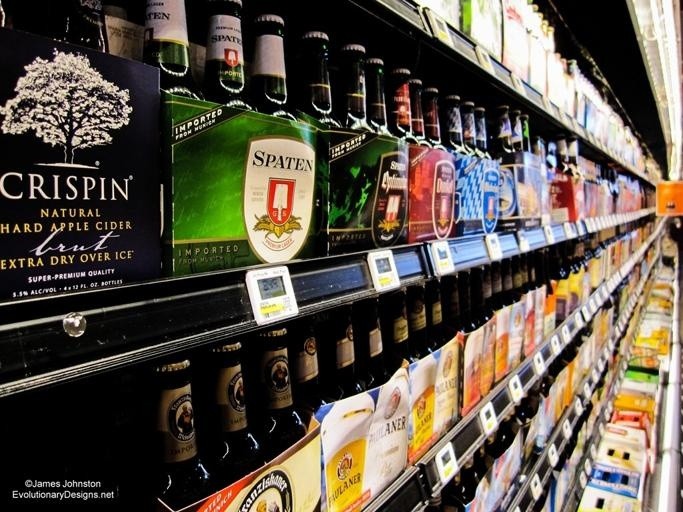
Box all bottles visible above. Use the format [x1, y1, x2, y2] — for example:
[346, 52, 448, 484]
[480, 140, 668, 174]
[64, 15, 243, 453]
[139, 0, 583, 512]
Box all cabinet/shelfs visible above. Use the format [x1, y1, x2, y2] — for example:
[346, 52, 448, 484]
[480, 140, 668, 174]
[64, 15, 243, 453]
[0, 0, 682, 512]
